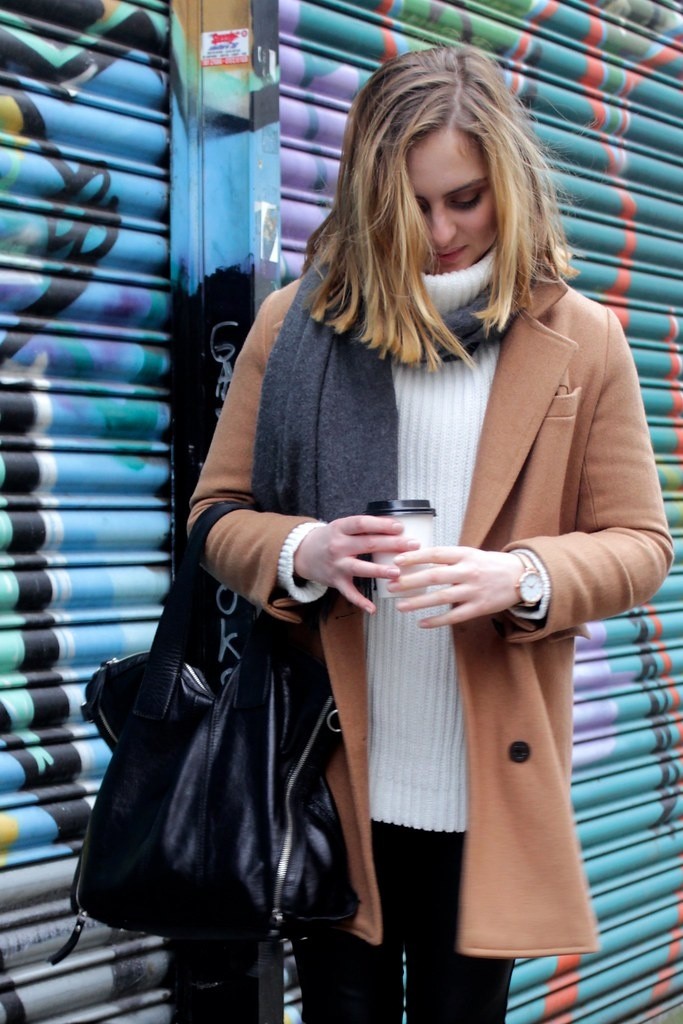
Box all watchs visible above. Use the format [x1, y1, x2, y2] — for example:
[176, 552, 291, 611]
[510, 549, 545, 609]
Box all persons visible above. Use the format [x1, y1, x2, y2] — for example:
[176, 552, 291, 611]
[184, 45, 676, 1024]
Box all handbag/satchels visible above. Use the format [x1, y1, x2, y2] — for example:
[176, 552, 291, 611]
[46, 506, 358, 965]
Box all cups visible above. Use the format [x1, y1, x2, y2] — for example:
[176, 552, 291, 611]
[363, 500, 436, 598]
